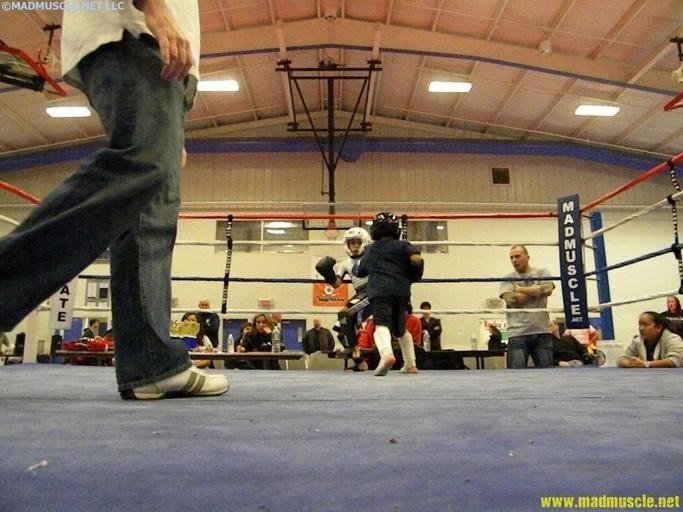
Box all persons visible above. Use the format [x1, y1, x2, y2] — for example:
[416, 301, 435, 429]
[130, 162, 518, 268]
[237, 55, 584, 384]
[0, 1, 231, 400]
[497, 244, 556, 368]
[313, 225, 375, 371]
[0, 295, 680, 369]
[350, 212, 425, 377]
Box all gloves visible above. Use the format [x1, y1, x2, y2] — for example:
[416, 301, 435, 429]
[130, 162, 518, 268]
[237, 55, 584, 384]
[316, 257, 336, 283]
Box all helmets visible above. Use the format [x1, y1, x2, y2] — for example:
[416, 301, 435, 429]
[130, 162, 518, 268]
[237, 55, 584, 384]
[344, 227, 370, 258]
[369, 212, 400, 240]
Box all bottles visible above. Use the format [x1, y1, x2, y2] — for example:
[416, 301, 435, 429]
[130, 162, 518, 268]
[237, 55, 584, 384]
[271, 326, 281, 352]
[470, 334, 477, 351]
[422, 331, 430, 352]
[226, 333, 234, 353]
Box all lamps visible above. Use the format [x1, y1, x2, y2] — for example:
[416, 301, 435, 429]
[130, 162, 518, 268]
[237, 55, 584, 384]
[320, 3, 342, 28]
[534, 20, 559, 59]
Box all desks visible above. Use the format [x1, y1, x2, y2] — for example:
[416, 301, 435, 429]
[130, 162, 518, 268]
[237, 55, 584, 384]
[52, 331, 510, 371]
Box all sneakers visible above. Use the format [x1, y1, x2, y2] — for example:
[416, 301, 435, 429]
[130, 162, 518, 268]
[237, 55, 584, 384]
[120, 365, 230, 400]
